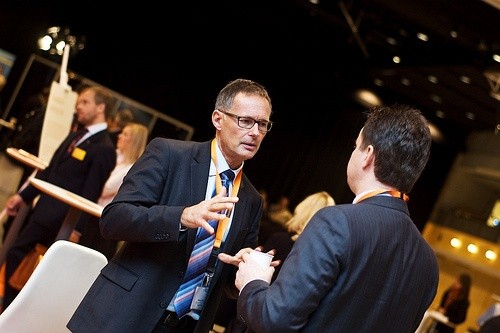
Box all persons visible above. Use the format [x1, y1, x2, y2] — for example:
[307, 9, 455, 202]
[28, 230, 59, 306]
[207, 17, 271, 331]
[0, 86, 116, 314]
[18, 87, 132, 191]
[69, 123, 148, 262]
[253, 187, 335, 290]
[433, 269, 474, 333]
[235, 106, 439, 333]
[66, 78, 281, 333]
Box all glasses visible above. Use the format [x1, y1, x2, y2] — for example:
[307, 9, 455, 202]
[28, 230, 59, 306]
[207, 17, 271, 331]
[218, 109, 272, 132]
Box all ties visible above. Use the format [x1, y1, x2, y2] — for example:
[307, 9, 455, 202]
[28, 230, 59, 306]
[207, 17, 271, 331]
[61, 128, 89, 159]
[173, 170, 235, 320]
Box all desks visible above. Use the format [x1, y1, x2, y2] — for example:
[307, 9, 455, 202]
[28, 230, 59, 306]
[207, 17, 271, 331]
[28, 176, 105, 241]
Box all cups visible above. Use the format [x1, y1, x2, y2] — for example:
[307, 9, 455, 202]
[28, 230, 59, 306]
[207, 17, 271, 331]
[249, 249, 274, 269]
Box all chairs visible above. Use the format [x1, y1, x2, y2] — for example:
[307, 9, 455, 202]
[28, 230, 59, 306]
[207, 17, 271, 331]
[0, 240, 109, 333]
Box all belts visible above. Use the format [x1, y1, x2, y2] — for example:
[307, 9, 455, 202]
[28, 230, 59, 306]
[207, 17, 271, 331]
[160, 311, 197, 329]
